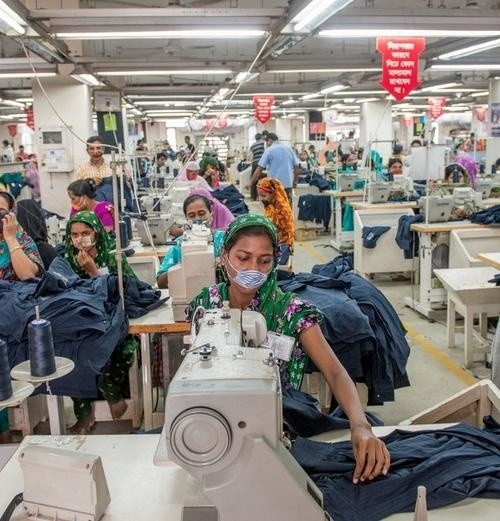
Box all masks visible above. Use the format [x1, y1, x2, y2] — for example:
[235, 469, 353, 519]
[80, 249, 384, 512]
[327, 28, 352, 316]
[224, 255, 272, 290]
[0, 209, 12, 219]
[87, 151, 103, 158]
[70, 230, 97, 250]
[262, 200, 269, 206]
[188, 175, 197, 180]
[393, 170, 403, 174]
[188, 214, 212, 225]
[72, 196, 86, 210]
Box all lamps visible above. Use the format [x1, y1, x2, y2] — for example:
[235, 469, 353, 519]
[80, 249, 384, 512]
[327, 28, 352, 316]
[0, 0, 500, 114]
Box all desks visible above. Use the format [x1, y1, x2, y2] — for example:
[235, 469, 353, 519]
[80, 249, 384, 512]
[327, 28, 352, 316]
[432, 265, 499, 370]
[406, 218, 485, 324]
[1, 418, 499, 521]
[121, 286, 202, 434]
[2, 158, 499, 278]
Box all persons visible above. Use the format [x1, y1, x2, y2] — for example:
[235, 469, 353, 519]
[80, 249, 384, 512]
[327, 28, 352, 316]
[299, 129, 500, 200]
[1, 135, 136, 433]
[187, 212, 391, 483]
[155, 195, 226, 289]
[136, 132, 301, 279]
